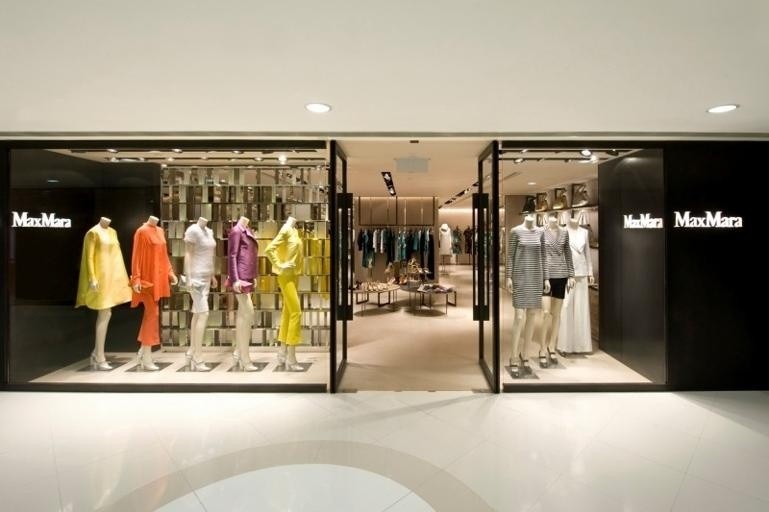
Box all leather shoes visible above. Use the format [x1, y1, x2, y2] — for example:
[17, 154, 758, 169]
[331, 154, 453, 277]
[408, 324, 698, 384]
[519, 353, 532, 374]
[538, 350, 548, 369]
[509, 358, 520, 378]
[547, 347, 558, 364]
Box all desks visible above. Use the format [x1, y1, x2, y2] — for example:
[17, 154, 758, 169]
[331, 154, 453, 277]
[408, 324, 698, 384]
[349, 281, 457, 316]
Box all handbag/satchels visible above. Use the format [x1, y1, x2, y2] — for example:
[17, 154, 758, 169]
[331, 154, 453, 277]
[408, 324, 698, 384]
[572, 187, 591, 208]
[178, 276, 208, 295]
[552, 191, 569, 209]
[225, 276, 253, 294]
[578, 211, 592, 239]
[536, 194, 551, 212]
[127, 275, 154, 295]
[522, 195, 536, 211]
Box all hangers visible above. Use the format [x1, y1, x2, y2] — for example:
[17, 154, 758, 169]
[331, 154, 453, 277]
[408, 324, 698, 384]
[360, 223, 430, 233]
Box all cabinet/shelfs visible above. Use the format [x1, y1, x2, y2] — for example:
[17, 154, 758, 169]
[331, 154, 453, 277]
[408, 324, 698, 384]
[518, 183, 599, 342]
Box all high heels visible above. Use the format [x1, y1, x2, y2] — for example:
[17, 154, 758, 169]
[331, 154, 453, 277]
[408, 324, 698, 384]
[233, 351, 258, 371]
[90, 353, 113, 370]
[186, 354, 210, 371]
[137, 352, 159, 370]
[277, 352, 304, 371]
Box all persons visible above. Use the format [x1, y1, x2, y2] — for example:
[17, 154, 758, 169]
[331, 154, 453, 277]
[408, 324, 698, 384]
[263, 216, 311, 371]
[437, 222, 454, 258]
[123, 214, 179, 370]
[451, 226, 464, 255]
[503, 212, 553, 379]
[181, 216, 220, 372]
[226, 214, 261, 370]
[557, 218, 596, 358]
[463, 226, 473, 255]
[72, 215, 134, 370]
[537, 210, 577, 369]
